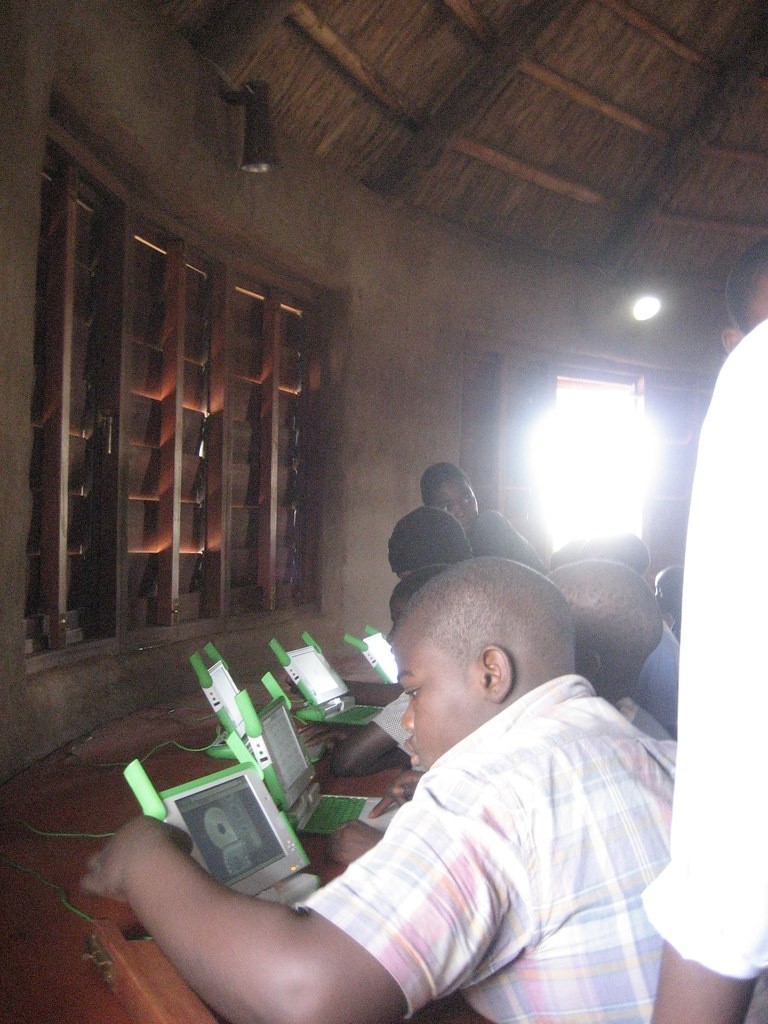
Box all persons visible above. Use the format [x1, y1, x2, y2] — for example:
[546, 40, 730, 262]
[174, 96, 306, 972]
[388, 463, 688, 743]
[79, 556, 674, 1024]
[640, 319, 768, 1024]
[721, 237, 768, 355]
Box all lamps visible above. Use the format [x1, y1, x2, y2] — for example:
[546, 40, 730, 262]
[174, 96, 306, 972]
[223, 79, 284, 173]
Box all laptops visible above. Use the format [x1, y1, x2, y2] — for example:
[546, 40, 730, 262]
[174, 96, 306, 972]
[124, 626, 399, 940]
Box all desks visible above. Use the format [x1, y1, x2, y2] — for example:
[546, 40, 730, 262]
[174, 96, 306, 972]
[0, 655, 407, 1024]
